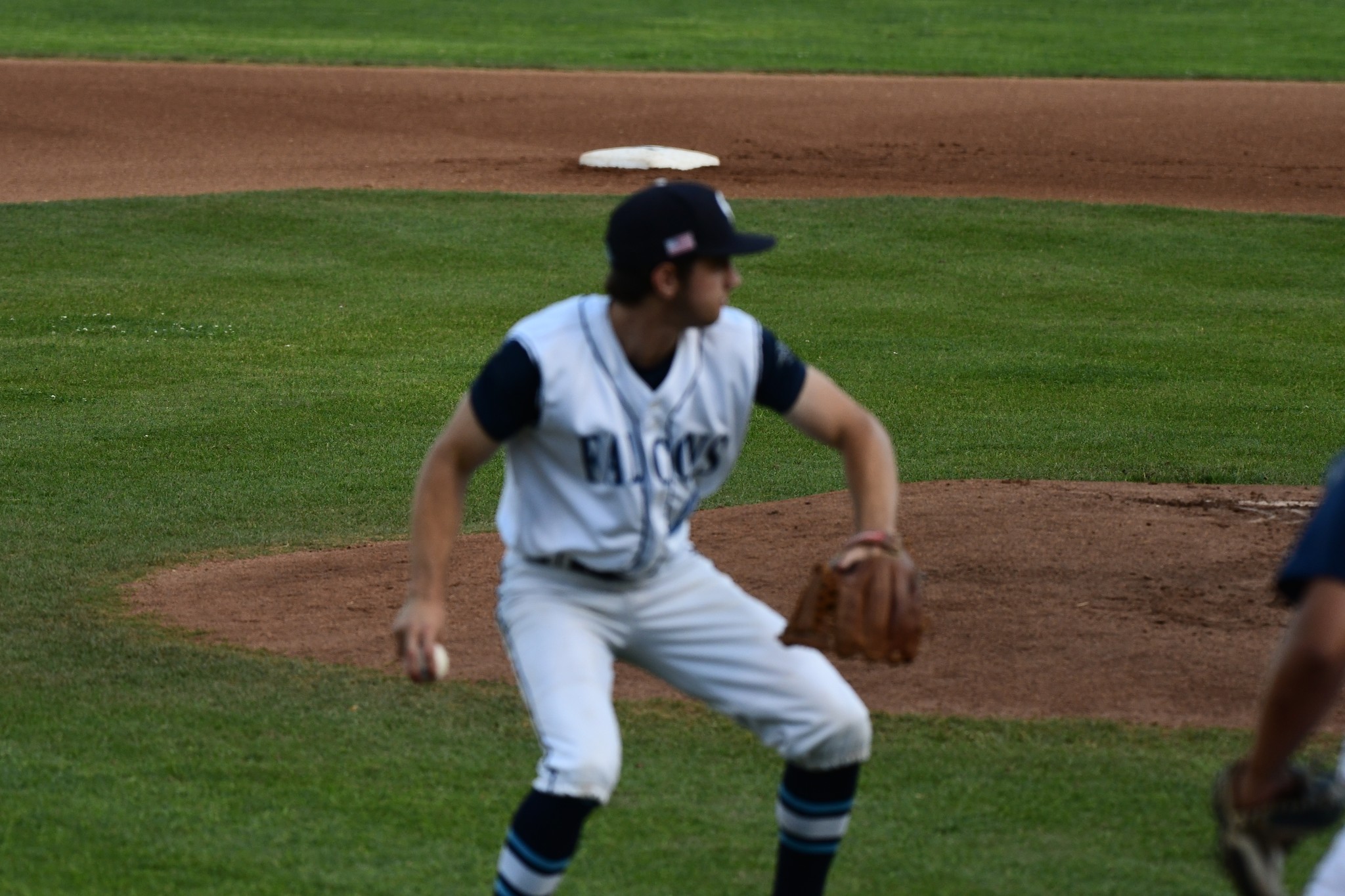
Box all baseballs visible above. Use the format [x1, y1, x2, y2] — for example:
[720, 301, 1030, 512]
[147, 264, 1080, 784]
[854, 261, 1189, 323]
[401, 642, 454, 686]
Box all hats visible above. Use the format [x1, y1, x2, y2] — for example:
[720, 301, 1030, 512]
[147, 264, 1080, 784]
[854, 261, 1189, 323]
[607, 180, 775, 263]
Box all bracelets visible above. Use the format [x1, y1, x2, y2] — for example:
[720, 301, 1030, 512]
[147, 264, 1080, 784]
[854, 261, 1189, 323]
[841, 527, 907, 554]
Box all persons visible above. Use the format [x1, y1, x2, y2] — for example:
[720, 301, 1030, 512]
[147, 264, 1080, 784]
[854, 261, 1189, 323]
[387, 176, 918, 896]
[1212, 444, 1345, 896]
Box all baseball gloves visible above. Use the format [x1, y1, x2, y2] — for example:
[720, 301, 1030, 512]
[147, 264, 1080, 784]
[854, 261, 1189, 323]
[1210, 756, 1344, 896]
[777, 532, 925, 672]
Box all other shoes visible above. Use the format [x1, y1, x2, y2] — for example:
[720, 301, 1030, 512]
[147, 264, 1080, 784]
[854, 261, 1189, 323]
[1213, 762, 1288, 896]
